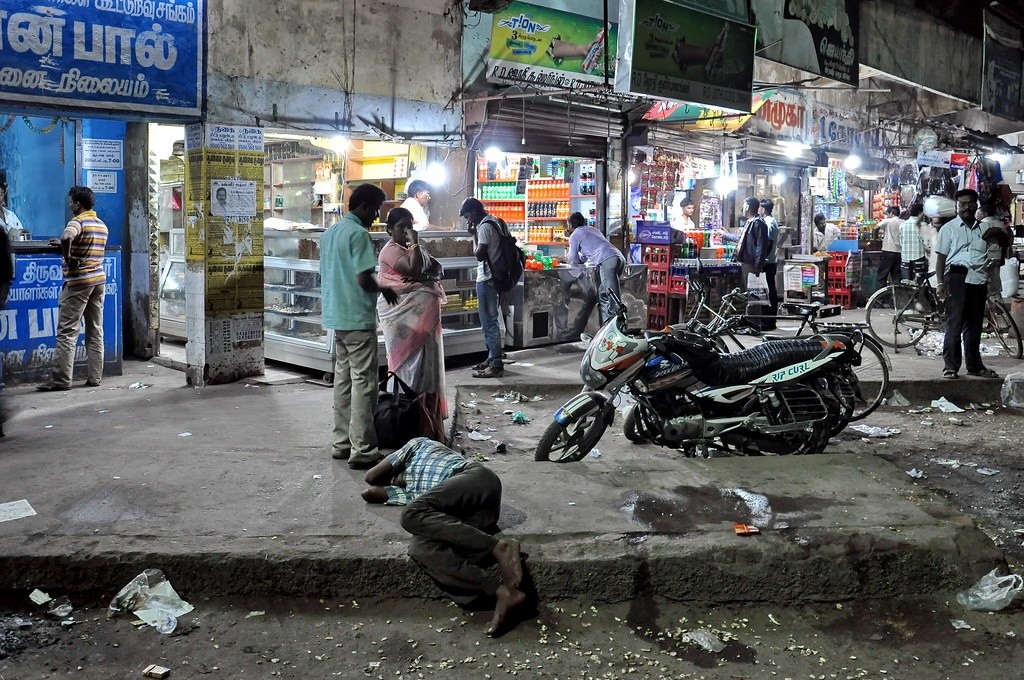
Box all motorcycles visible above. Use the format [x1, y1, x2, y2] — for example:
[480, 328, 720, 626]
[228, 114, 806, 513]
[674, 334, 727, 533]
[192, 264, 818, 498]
[624, 257, 865, 445]
[533, 288, 848, 463]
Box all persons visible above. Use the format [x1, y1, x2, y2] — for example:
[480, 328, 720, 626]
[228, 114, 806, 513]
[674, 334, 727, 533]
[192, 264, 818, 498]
[377, 208, 449, 421]
[718, 197, 768, 337]
[0, 183, 23, 233]
[898, 203, 927, 309]
[874, 207, 905, 310]
[37, 186, 108, 391]
[400, 180, 453, 231]
[554, 212, 626, 328]
[758, 198, 779, 332]
[362, 437, 526, 635]
[672, 197, 695, 230]
[975, 205, 1003, 333]
[935, 189, 1010, 378]
[553, 39, 603, 62]
[459, 197, 504, 377]
[555, 270, 598, 339]
[213, 188, 230, 213]
[320, 183, 399, 469]
[915, 211, 954, 288]
[813, 213, 840, 251]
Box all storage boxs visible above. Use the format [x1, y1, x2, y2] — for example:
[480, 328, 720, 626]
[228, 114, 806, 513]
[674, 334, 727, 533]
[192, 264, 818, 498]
[827, 252, 857, 310]
[643, 244, 686, 332]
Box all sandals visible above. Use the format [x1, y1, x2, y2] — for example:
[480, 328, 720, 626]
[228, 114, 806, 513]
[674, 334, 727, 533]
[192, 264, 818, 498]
[942, 367, 959, 378]
[966, 370, 1000, 378]
[471, 359, 490, 369]
[471, 364, 504, 378]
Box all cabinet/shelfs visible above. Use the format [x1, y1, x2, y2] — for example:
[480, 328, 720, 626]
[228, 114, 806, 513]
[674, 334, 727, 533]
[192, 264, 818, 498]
[815, 201, 848, 226]
[264, 137, 427, 229]
[477, 160, 598, 246]
[158, 228, 504, 382]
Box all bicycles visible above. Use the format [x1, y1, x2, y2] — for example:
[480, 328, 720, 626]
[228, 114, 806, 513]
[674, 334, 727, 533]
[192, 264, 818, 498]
[697, 287, 892, 424]
[865, 255, 1022, 359]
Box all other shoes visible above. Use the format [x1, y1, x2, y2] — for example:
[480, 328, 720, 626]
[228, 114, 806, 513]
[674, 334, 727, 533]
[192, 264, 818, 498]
[737, 327, 761, 334]
[36, 382, 71, 393]
[86, 379, 101, 386]
[349, 455, 385, 469]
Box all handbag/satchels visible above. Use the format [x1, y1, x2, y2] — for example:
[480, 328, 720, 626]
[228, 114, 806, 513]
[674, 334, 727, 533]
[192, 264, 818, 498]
[746, 272, 772, 307]
[374, 371, 422, 449]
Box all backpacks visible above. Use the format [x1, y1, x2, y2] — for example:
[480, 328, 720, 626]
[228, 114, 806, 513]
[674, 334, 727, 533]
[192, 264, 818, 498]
[474, 218, 526, 293]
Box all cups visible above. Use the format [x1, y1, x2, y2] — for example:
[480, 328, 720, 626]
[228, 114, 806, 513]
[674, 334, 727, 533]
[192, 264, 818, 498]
[438, 278, 478, 315]
[369, 193, 406, 232]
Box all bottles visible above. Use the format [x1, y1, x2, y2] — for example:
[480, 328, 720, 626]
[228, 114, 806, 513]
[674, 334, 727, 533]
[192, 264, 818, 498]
[478, 151, 596, 268]
[810, 166, 900, 308]
[264, 143, 343, 207]
[642, 228, 740, 329]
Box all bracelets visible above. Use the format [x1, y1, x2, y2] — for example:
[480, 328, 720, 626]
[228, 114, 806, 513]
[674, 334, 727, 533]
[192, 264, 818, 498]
[937, 282, 943, 286]
[411, 244, 418, 249]
